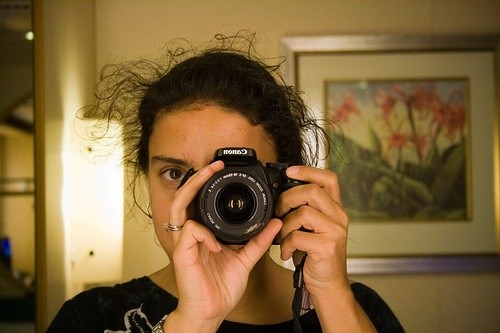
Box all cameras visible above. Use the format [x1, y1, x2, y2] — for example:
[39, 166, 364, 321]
[179, 148, 308, 249]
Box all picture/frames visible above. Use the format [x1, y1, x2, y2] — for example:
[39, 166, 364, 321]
[277, 31, 500, 278]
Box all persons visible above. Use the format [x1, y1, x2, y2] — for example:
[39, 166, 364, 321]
[43, 29, 408, 333]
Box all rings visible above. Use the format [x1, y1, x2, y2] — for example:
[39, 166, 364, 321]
[165, 223, 184, 232]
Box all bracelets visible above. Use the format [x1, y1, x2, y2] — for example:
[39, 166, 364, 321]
[152, 319, 166, 333]
